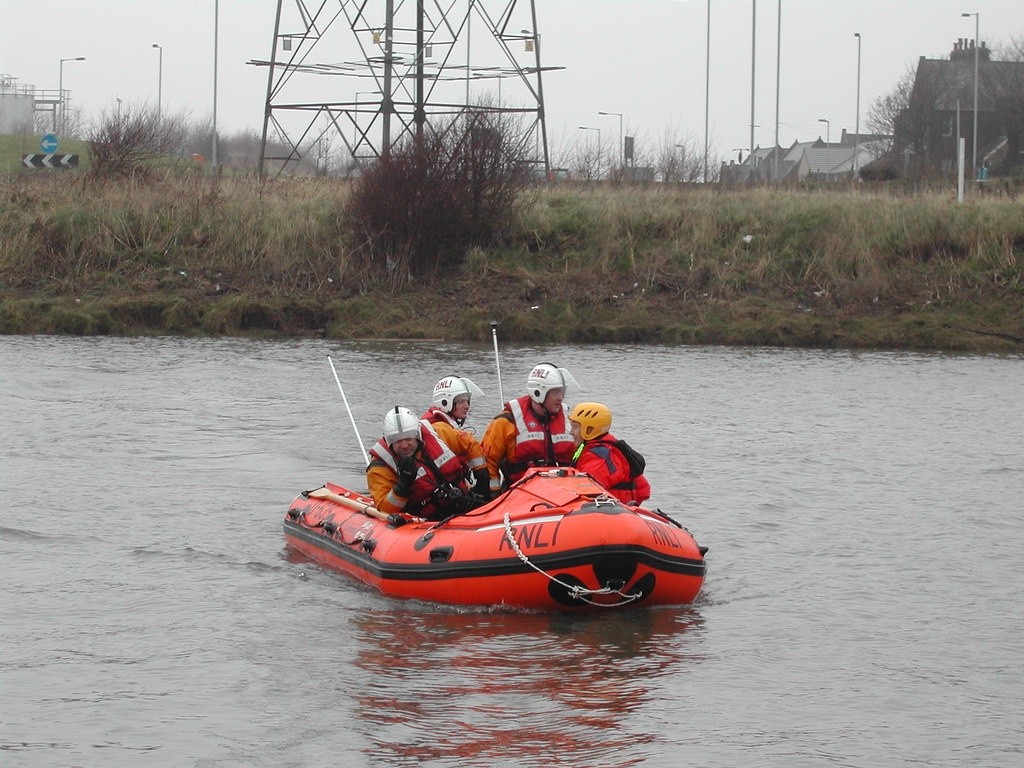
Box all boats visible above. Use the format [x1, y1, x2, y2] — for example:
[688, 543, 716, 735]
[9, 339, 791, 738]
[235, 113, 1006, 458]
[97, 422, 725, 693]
[282, 466, 708, 612]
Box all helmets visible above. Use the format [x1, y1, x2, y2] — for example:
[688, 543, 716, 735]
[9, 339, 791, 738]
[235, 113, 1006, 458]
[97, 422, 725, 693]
[433, 375, 470, 413]
[527, 362, 568, 404]
[569, 402, 612, 440]
[383, 406, 421, 442]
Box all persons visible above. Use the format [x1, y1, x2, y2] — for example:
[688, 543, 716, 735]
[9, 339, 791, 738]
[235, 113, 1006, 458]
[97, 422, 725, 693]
[364, 405, 491, 523]
[568, 402, 650, 507]
[475, 361, 584, 501]
[418, 375, 493, 429]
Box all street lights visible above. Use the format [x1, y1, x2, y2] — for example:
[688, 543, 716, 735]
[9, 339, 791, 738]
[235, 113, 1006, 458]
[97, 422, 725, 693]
[521, 29, 541, 170]
[732, 148, 750, 164]
[579, 127, 602, 174]
[60, 56, 85, 115]
[819, 119, 829, 183]
[854, 33, 861, 176]
[961, 12, 978, 180]
[676, 144, 685, 182]
[599, 112, 622, 173]
[153, 44, 162, 157]
[117, 97, 121, 126]
[353, 91, 381, 151]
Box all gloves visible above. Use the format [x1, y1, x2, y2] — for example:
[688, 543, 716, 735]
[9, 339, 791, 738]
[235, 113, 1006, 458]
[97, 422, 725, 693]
[393, 456, 419, 498]
[469, 468, 491, 496]
